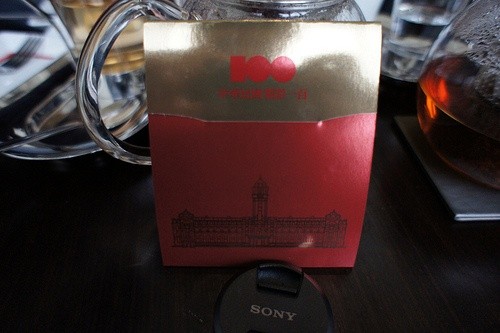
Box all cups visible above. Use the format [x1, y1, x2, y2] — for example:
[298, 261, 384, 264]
[381, 0, 471, 82]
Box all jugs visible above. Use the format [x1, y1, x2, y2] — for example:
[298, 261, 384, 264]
[76, 0, 367, 166]
[416, 0, 500, 188]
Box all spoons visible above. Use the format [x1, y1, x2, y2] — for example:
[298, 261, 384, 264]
[0, 98, 141, 153]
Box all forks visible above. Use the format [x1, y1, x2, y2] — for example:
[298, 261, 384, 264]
[0, 37, 43, 76]
